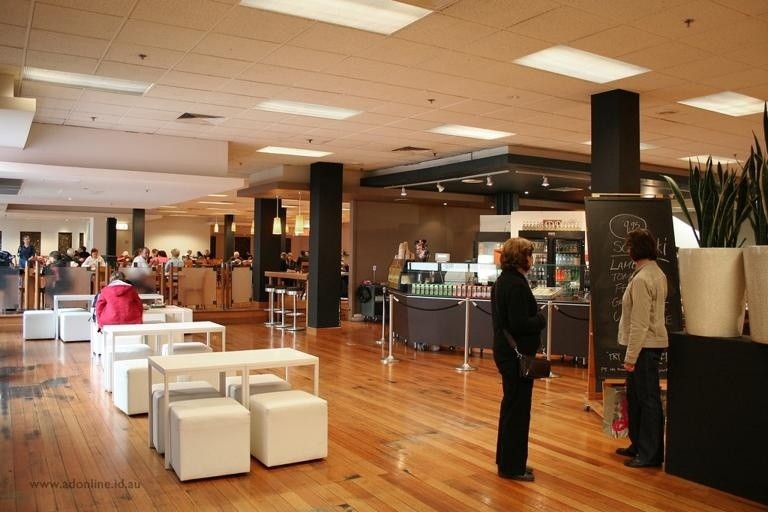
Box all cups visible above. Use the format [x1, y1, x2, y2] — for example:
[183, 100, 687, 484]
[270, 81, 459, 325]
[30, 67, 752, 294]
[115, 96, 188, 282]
[425, 278, 431, 283]
[155, 296, 164, 304]
[570, 281, 579, 300]
[395, 241, 415, 259]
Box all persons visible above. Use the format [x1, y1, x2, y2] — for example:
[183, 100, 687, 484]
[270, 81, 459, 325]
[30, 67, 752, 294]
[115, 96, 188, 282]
[0, 234, 310, 302]
[489, 236, 547, 481]
[95, 271, 144, 330]
[615, 228, 670, 472]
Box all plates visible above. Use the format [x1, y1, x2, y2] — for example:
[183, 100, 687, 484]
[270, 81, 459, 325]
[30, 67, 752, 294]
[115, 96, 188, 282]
[150, 305, 166, 308]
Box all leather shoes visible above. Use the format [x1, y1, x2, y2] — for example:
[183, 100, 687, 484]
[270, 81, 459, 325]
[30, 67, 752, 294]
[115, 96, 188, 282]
[498, 466, 535, 482]
[616, 447, 636, 458]
[624, 455, 663, 467]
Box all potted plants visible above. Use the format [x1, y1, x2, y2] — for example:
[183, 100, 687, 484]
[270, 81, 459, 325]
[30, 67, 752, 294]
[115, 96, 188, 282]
[661, 158, 748, 338]
[743, 101, 768, 345]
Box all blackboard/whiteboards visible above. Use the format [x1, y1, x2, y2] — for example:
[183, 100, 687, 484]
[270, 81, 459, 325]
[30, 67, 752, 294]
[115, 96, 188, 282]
[583, 197, 683, 401]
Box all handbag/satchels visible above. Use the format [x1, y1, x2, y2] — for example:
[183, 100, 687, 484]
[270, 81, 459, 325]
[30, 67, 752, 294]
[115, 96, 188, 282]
[518, 354, 552, 380]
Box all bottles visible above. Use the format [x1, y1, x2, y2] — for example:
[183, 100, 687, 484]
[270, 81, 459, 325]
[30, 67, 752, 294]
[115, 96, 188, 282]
[532, 237, 548, 252]
[526, 266, 545, 279]
[528, 280, 547, 288]
[556, 254, 581, 264]
[522, 220, 578, 231]
[556, 244, 580, 253]
[535, 255, 548, 264]
[555, 267, 580, 281]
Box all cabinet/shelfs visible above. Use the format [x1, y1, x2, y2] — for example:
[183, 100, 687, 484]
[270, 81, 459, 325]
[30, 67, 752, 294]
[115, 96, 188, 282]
[359, 285, 388, 322]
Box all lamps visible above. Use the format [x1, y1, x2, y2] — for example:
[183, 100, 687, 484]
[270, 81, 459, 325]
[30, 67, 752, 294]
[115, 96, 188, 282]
[516, 170, 591, 187]
[214, 195, 304, 235]
[384, 169, 509, 196]
